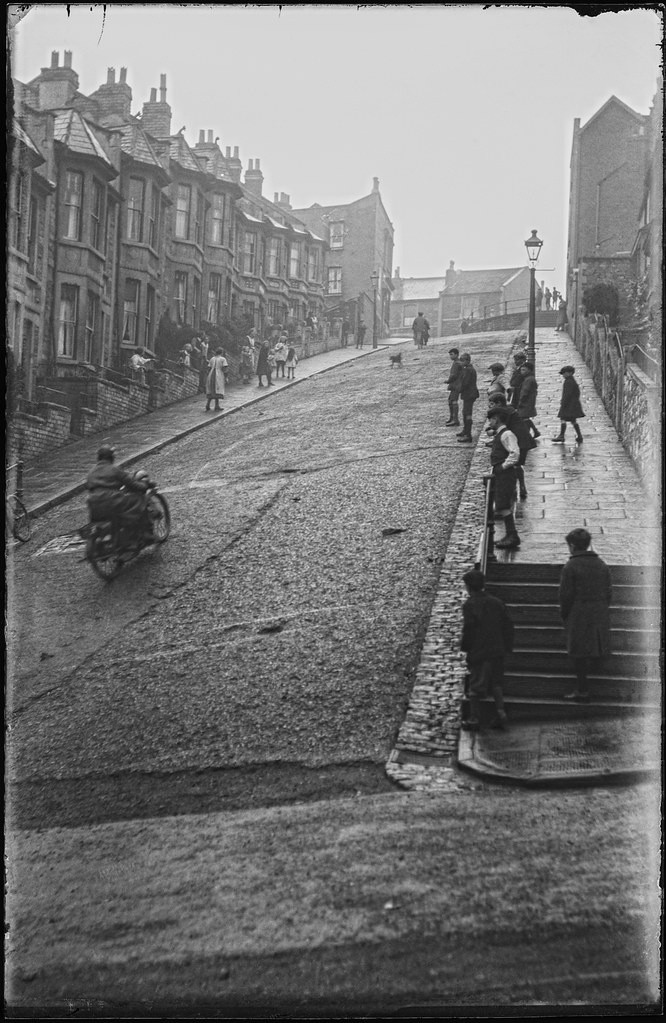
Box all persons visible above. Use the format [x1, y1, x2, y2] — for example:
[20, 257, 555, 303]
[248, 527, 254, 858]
[555, 295, 567, 331]
[177, 331, 227, 410]
[413, 312, 430, 341]
[487, 392, 536, 498]
[559, 527, 618, 695]
[536, 287, 560, 311]
[356, 320, 366, 349]
[551, 366, 586, 442]
[240, 332, 298, 385]
[456, 353, 479, 442]
[487, 408, 521, 549]
[459, 571, 512, 729]
[129, 348, 152, 369]
[306, 312, 317, 330]
[444, 349, 462, 426]
[488, 351, 540, 439]
[87, 448, 148, 552]
[341, 319, 350, 349]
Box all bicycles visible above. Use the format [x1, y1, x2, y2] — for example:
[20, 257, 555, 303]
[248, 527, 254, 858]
[5, 494, 31, 542]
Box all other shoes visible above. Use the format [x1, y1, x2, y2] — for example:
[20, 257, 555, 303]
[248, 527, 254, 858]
[446, 418, 460, 426]
[206, 405, 210, 411]
[215, 407, 224, 412]
[457, 430, 471, 437]
[268, 383, 275, 386]
[488, 715, 507, 728]
[576, 437, 583, 443]
[462, 717, 481, 728]
[457, 434, 472, 443]
[494, 532, 520, 548]
[565, 690, 591, 704]
[552, 437, 564, 442]
[259, 383, 263, 386]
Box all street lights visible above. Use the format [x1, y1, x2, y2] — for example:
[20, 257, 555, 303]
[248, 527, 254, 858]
[369, 270, 379, 348]
[524, 229, 543, 366]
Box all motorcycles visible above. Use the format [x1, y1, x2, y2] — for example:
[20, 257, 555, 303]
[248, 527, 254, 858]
[77, 469, 171, 581]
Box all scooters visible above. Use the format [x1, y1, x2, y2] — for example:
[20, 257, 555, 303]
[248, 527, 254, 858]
[412, 326, 430, 349]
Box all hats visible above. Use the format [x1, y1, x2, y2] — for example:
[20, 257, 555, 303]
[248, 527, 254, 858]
[560, 366, 575, 374]
[486, 407, 508, 418]
